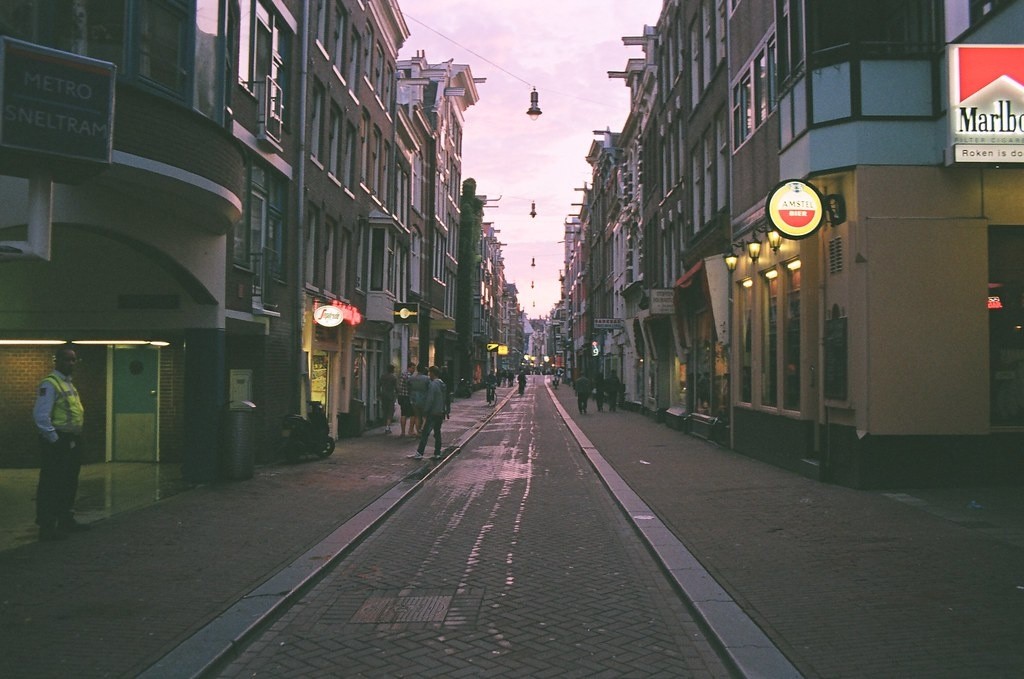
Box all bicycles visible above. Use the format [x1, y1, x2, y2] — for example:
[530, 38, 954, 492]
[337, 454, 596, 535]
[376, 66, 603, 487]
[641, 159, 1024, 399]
[488, 382, 497, 407]
[519, 379, 525, 396]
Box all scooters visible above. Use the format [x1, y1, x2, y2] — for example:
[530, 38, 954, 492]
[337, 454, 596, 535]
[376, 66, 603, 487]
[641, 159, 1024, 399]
[550, 374, 561, 390]
[279, 399, 336, 462]
[460, 375, 479, 398]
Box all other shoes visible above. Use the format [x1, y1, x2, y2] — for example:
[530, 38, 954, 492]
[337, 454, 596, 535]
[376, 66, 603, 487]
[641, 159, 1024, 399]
[428, 454, 441, 460]
[385, 427, 392, 434]
[412, 451, 424, 458]
[408, 432, 415, 437]
[39, 527, 69, 541]
[58, 519, 91, 533]
[400, 432, 406, 438]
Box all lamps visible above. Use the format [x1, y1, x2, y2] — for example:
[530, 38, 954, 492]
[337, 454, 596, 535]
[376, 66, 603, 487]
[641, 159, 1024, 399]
[747, 224, 769, 264]
[762, 222, 785, 255]
[526, 86, 543, 123]
[529, 257, 536, 308]
[722, 237, 745, 274]
[530, 202, 537, 219]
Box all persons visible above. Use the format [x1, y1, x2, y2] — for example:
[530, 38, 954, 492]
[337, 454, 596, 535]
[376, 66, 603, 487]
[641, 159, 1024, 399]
[32, 349, 91, 542]
[499, 365, 514, 387]
[517, 371, 527, 394]
[592, 369, 619, 412]
[377, 365, 397, 434]
[485, 369, 497, 402]
[398, 362, 447, 460]
[575, 371, 592, 414]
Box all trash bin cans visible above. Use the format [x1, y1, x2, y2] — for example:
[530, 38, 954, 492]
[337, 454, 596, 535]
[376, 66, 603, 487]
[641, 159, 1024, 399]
[221, 399, 255, 481]
